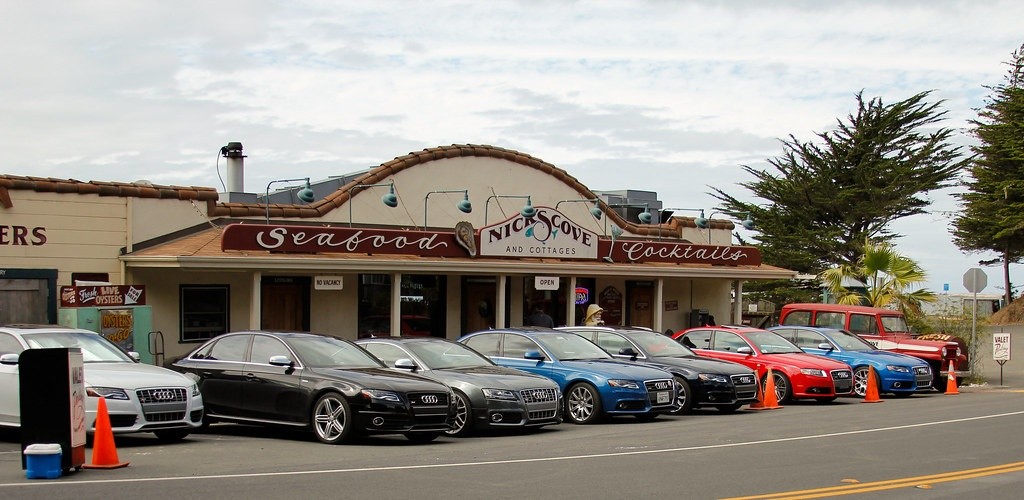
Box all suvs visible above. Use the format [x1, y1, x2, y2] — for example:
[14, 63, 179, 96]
[777, 302, 972, 394]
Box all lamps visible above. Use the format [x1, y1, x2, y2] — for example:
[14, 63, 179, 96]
[424, 190, 472, 231]
[349, 182, 398, 227]
[556, 198, 602, 220]
[267, 178, 314, 224]
[485, 195, 537, 226]
[659, 208, 706, 242]
[709, 211, 755, 245]
[605, 202, 651, 239]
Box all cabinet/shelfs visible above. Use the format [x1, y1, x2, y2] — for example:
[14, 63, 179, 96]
[184, 312, 224, 331]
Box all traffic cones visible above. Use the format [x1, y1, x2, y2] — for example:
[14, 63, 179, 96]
[943, 360, 960, 395]
[859, 362, 885, 403]
[82, 396, 131, 470]
[742, 370, 771, 410]
[763, 367, 785, 410]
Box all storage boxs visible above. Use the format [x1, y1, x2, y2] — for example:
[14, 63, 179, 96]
[24, 444, 62, 479]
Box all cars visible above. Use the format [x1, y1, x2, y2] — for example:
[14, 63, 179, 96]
[754, 325, 934, 399]
[325, 333, 565, 439]
[166, 329, 459, 446]
[438, 325, 679, 426]
[647, 325, 856, 407]
[0, 323, 205, 450]
[536, 324, 760, 417]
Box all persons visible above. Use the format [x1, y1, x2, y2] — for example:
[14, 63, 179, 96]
[527, 302, 554, 327]
[586, 303, 604, 325]
